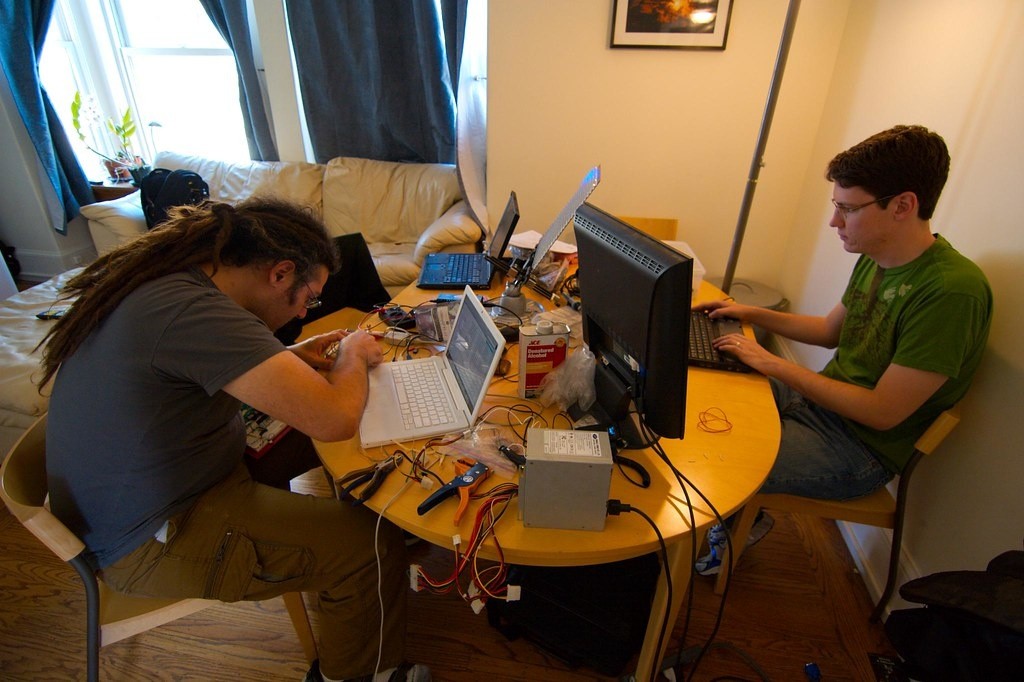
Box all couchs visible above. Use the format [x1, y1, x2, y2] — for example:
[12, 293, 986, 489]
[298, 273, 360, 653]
[0, 263, 89, 461]
[79, 151, 481, 300]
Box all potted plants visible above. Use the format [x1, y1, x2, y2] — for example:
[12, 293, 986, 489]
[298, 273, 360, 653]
[72, 91, 141, 177]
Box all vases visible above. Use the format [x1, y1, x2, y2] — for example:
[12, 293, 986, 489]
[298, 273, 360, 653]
[128, 166, 152, 184]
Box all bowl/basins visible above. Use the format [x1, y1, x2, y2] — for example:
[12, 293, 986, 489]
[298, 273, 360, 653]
[660, 240, 706, 292]
[508, 230, 577, 263]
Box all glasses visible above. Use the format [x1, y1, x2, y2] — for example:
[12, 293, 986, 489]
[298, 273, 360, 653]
[297, 270, 322, 310]
[831, 195, 895, 223]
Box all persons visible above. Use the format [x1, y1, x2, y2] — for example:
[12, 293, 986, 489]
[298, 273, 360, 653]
[694, 124, 994, 575]
[30, 197, 430, 682]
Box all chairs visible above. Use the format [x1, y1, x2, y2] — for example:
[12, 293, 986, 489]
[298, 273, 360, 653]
[0, 412, 319, 682]
[717, 404, 962, 624]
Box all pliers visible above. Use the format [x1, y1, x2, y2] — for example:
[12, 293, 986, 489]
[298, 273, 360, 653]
[336, 454, 402, 506]
[417, 457, 494, 526]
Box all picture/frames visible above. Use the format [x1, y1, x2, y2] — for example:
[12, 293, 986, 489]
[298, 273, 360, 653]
[609, 0, 734, 51]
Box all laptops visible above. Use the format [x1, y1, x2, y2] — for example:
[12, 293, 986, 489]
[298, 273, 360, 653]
[532, 165, 601, 277]
[415, 191, 520, 289]
[358, 284, 506, 450]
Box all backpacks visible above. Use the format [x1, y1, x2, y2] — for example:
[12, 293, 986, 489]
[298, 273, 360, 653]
[140, 169, 210, 230]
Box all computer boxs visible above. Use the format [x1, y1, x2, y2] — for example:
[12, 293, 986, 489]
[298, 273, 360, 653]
[484, 552, 662, 679]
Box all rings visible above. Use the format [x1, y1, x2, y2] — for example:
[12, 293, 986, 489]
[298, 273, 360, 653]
[736, 342, 740, 346]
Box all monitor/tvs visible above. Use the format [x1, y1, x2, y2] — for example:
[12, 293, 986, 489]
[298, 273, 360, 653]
[567, 202, 694, 450]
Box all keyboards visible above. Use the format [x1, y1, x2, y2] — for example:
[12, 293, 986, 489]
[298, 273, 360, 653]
[688, 310, 753, 374]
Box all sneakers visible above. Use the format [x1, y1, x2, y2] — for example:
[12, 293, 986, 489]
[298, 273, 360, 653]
[695, 511, 775, 577]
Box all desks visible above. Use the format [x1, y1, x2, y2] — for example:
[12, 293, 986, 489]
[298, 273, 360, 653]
[309, 271, 784, 682]
